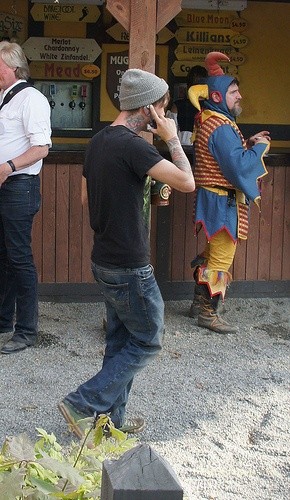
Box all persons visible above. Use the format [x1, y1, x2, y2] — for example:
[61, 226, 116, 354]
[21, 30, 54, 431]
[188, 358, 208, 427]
[185, 52, 272, 333]
[173, 65, 208, 146]
[56, 69, 195, 449]
[0, 41, 53, 354]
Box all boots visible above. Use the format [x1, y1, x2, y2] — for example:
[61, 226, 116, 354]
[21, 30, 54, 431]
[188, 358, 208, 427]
[190, 285, 207, 318]
[198, 285, 239, 333]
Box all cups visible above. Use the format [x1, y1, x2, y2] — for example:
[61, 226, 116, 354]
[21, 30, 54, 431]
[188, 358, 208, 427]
[151, 179, 170, 206]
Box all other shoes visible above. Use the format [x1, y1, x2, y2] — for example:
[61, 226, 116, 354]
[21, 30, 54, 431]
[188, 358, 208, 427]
[58, 401, 97, 449]
[1, 340, 28, 354]
[119, 417, 145, 434]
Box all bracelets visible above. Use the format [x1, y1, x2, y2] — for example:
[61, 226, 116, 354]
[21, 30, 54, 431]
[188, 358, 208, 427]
[7, 160, 16, 172]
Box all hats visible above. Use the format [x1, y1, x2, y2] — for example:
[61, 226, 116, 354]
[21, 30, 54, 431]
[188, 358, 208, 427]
[118, 67, 169, 111]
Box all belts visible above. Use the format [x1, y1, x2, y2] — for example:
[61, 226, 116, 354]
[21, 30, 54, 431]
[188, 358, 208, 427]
[6, 174, 38, 181]
